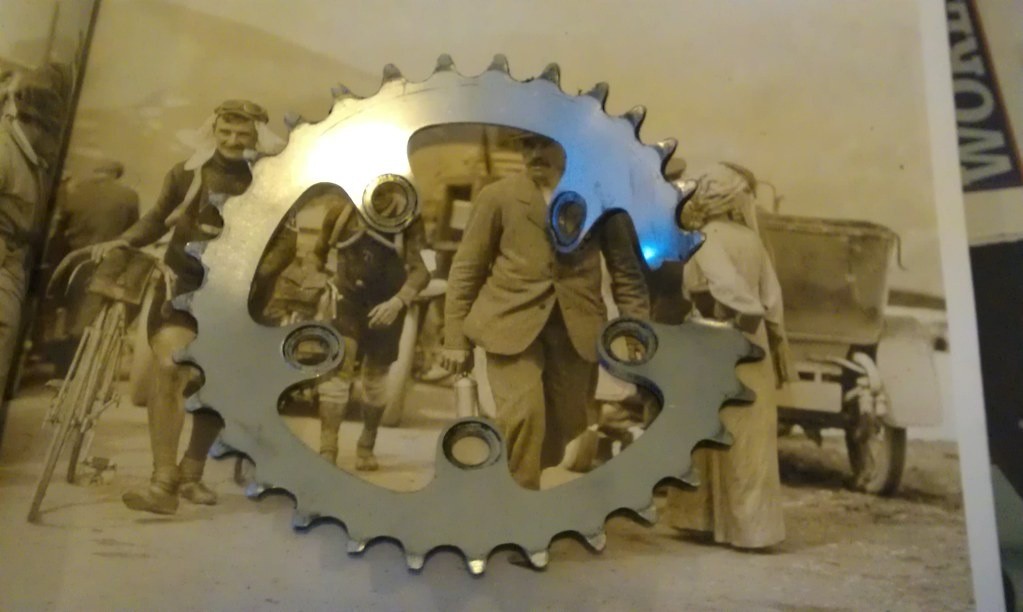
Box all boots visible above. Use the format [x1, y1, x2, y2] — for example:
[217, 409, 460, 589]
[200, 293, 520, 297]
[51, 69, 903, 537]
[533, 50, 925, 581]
[179, 455, 216, 506]
[353, 427, 377, 468]
[121, 465, 180, 515]
[317, 427, 339, 468]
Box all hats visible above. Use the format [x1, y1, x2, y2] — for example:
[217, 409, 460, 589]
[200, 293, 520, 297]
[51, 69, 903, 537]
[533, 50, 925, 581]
[215, 100, 269, 125]
[15, 61, 72, 133]
[692, 163, 758, 213]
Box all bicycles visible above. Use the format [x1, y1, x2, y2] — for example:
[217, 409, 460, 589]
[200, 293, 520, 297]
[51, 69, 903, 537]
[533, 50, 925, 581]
[272, 264, 336, 415]
[22, 241, 182, 524]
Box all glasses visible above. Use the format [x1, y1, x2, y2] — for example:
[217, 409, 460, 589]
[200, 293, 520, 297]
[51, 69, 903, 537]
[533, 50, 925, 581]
[221, 102, 265, 116]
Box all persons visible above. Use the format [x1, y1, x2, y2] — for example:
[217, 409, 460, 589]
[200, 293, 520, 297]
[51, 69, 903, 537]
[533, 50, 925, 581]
[632, 155, 796, 552]
[437, 125, 656, 563]
[312, 180, 431, 474]
[49, 156, 141, 389]
[0, 65, 64, 397]
[91, 97, 298, 514]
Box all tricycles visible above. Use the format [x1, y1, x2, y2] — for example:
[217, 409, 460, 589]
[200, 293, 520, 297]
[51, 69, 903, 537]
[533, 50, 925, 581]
[566, 205, 909, 502]
